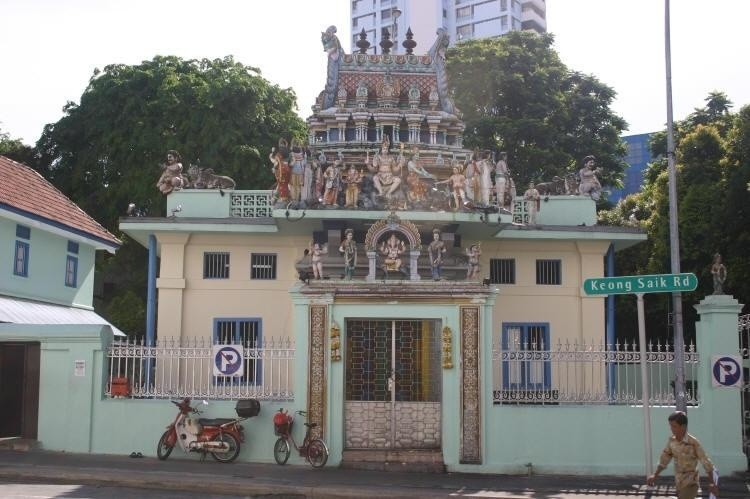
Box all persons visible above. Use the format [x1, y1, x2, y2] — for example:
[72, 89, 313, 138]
[578, 156, 601, 194]
[648, 412, 718, 498]
[711, 252, 727, 295]
[268, 138, 540, 280]
[155, 150, 184, 194]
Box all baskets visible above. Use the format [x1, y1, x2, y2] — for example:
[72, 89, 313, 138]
[274, 423, 290, 435]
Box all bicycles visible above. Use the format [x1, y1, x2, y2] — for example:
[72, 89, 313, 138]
[272, 408, 329, 468]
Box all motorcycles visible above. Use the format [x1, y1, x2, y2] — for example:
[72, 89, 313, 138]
[157, 388, 259, 464]
